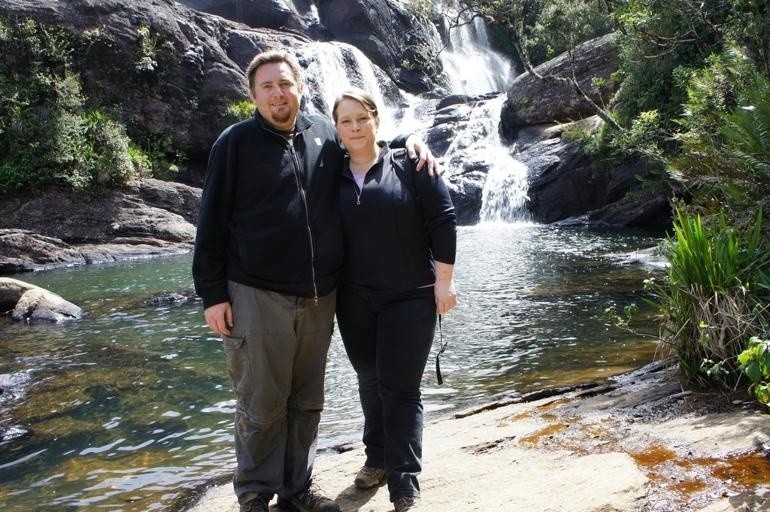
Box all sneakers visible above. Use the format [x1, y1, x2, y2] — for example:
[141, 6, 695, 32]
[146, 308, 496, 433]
[394, 496, 418, 512]
[355, 465, 386, 488]
[277, 481, 340, 512]
[238, 491, 269, 512]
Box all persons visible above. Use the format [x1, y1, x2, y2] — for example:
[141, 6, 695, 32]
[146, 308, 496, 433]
[318, 90, 459, 512]
[192, 48, 443, 511]
